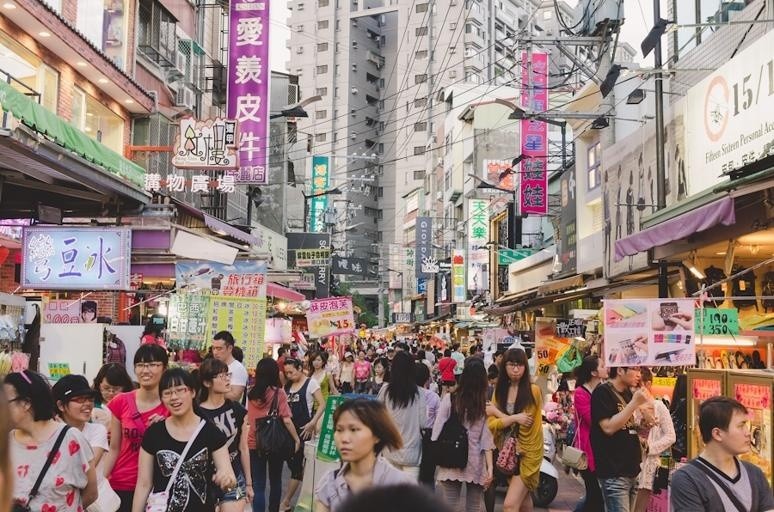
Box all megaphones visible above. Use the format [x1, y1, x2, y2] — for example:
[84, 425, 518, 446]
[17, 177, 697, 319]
[249, 184, 265, 209]
[246, 187, 262, 201]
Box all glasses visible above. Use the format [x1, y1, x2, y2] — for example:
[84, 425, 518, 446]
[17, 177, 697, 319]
[136, 362, 163, 368]
[68, 396, 94, 404]
[160, 386, 191, 398]
[211, 372, 234, 379]
[505, 362, 525, 369]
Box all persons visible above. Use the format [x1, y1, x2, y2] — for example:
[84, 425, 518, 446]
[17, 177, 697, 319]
[668, 313, 693, 329]
[0, 322, 773, 511]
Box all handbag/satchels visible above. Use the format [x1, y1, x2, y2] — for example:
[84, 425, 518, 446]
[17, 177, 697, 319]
[495, 434, 519, 476]
[556, 353, 583, 374]
[561, 446, 588, 471]
[11, 502, 30, 512]
[83, 474, 122, 512]
[145, 484, 170, 512]
[429, 381, 439, 394]
[254, 415, 298, 463]
[436, 411, 470, 469]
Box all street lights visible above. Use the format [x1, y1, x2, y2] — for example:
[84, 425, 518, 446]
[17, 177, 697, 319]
[302, 180, 351, 231]
[428, 243, 452, 254]
[467, 171, 517, 258]
[244, 94, 325, 235]
[382, 266, 405, 313]
[491, 97, 570, 327]
[331, 221, 364, 236]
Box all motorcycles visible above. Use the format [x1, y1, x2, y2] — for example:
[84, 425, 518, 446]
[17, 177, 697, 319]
[529, 409, 558, 507]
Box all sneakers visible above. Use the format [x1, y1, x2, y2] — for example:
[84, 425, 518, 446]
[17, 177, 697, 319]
[276, 502, 294, 512]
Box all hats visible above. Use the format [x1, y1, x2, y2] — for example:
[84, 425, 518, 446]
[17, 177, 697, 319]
[51, 373, 95, 403]
[345, 351, 352, 358]
